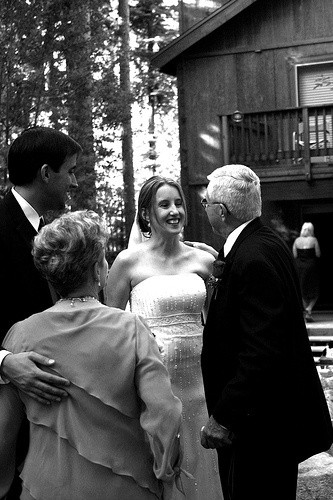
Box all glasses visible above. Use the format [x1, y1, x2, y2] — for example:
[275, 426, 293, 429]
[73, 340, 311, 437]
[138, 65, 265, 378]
[201, 197, 231, 216]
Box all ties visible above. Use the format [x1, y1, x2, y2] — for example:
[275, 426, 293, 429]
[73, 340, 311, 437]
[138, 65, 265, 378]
[38, 218, 46, 233]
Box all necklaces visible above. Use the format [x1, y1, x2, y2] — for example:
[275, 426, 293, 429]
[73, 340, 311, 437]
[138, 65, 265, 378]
[55, 297, 98, 307]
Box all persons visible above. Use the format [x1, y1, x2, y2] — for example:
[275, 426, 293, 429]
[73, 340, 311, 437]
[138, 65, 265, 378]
[293, 221, 324, 317]
[1, 124, 85, 408]
[200, 165, 333, 500]
[1, 208, 196, 500]
[104, 176, 224, 500]
